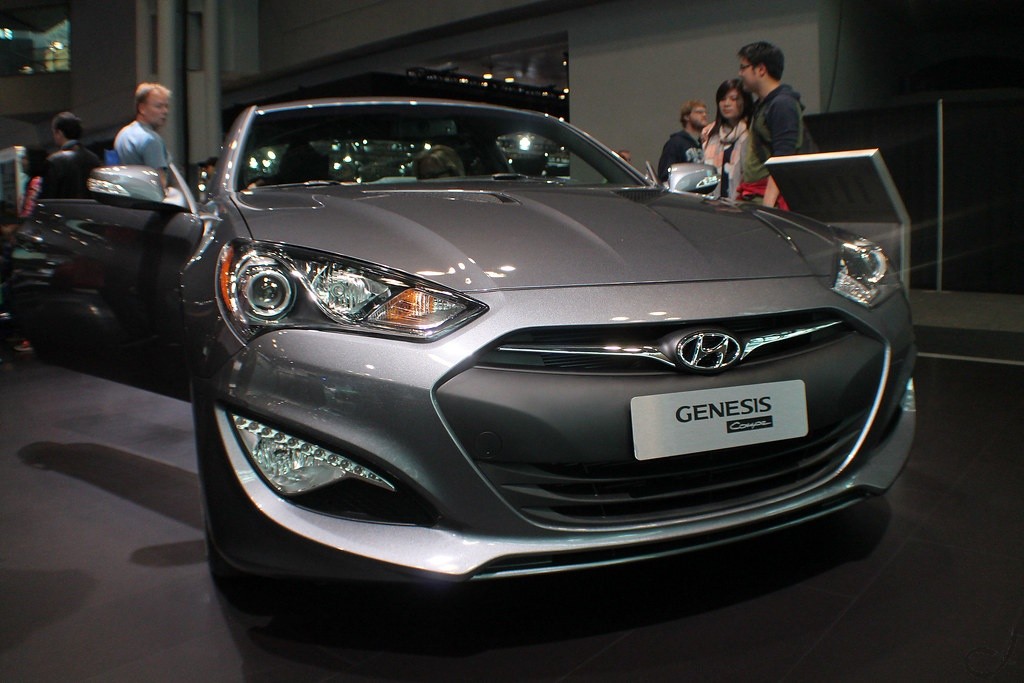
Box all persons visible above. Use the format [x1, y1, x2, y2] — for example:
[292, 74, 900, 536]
[604, 150, 631, 184]
[198, 156, 218, 185]
[114, 83, 174, 197]
[0, 112, 102, 350]
[658, 42, 807, 210]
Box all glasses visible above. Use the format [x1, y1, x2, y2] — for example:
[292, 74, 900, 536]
[740, 64, 753, 70]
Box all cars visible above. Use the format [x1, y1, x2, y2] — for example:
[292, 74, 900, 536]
[5, 94, 918, 606]
